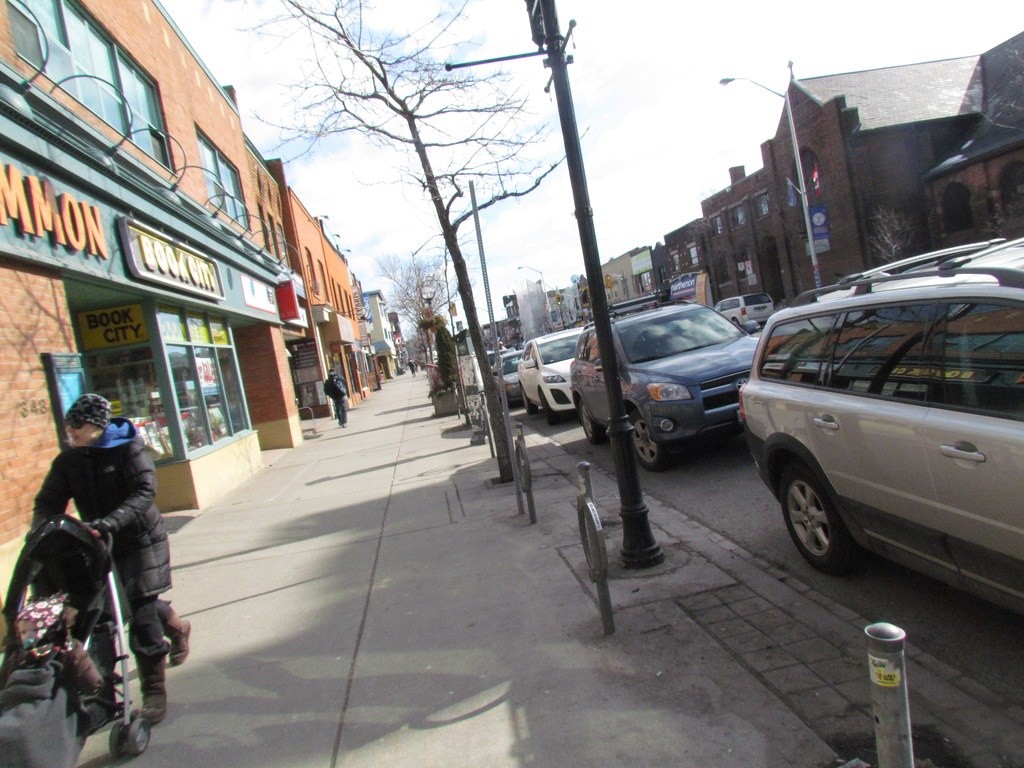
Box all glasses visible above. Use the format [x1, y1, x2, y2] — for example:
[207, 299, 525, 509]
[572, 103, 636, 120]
[68, 418, 87, 429]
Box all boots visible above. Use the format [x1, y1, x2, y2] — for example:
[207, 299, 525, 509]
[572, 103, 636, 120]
[165, 607, 192, 667]
[135, 654, 168, 724]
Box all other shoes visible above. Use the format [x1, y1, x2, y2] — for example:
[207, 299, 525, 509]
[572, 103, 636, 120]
[338, 422, 348, 428]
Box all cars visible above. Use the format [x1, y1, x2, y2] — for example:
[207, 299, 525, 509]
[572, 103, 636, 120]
[492, 350, 526, 407]
[487, 349, 511, 368]
[516, 327, 586, 425]
[790, 268, 893, 307]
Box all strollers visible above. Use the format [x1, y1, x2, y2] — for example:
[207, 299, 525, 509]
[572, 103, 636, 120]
[0, 515, 150, 767]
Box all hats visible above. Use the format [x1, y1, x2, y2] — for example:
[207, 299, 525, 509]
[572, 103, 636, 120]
[65, 393, 111, 428]
[328, 367, 337, 376]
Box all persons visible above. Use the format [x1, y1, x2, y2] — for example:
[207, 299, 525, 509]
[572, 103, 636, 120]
[0, 593, 102, 768]
[25, 394, 191, 727]
[494, 340, 506, 350]
[406, 357, 419, 377]
[323, 368, 348, 428]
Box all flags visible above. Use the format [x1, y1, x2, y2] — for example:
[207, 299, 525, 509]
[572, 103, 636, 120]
[783, 176, 797, 207]
[810, 165, 823, 197]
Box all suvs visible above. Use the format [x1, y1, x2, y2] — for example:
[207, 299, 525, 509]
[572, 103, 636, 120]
[568, 292, 760, 473]
[714, 293, 776, 332]
[736, 237, 1024, 616]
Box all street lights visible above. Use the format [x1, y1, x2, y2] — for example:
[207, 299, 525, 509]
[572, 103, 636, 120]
[518, 265, 555, 333]
[409, 231, 444, 363]
[713, 74, 823, 285]
